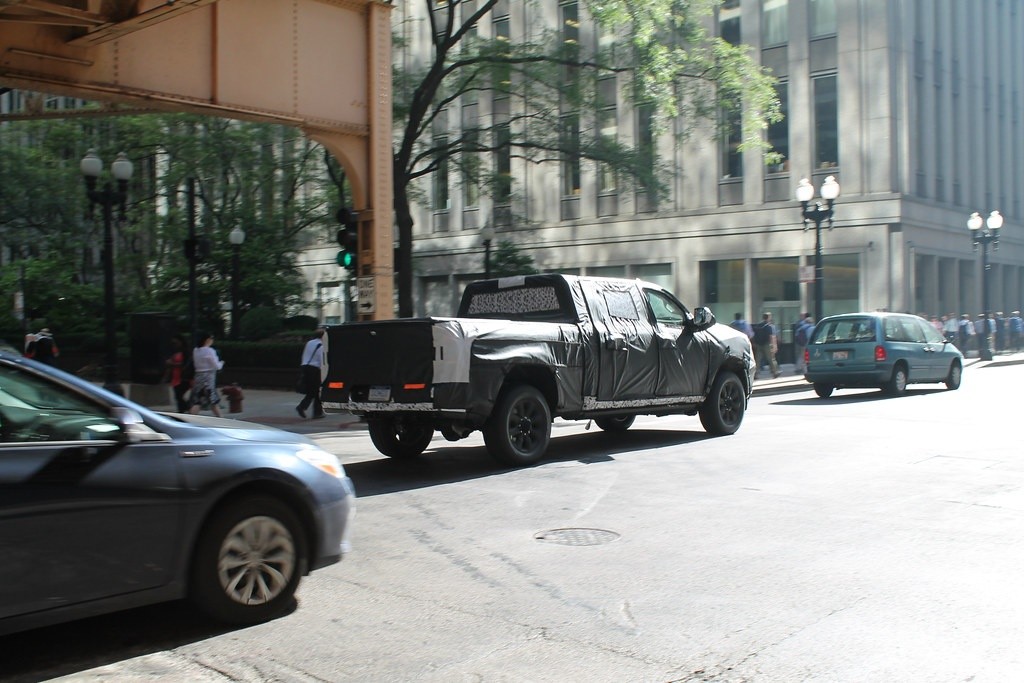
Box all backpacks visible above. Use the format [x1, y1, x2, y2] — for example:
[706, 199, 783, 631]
[797, 329, 808, 346]
[754, 322, 772, 346]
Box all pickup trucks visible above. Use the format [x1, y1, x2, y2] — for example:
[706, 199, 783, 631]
[319, 273, 755, 472]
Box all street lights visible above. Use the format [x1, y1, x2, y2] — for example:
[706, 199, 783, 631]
[230, 224, 247, 330]
[81, 147, 135, 399]
[481, 223, 493, 279]
[796, 176, 840, 326]
[967, 210, 1004, 360]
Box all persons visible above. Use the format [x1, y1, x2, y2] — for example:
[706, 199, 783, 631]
[168, 333, 225, 418]
[792, 312, 816, 377]
[24, 328, 58, 365]
[729, 312, 747, 334]
[751, 312, 783, 380]
[921, 311, 1024, 362]
[296, 329, 327, 419]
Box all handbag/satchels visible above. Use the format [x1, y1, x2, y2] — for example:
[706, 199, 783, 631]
[296, 372, 307, 394]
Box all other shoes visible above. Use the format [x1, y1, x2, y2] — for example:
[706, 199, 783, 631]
[774, 370, 783, 378]
[296, 404, 307, 419]
[312, 412, 330, 419]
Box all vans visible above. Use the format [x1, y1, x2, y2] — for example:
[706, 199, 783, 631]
[803, 312, 963, 398]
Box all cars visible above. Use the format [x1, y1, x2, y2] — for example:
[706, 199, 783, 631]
[0, 343, 358, 632]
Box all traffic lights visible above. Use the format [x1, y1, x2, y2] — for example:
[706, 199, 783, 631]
[336, 209, 357, 270]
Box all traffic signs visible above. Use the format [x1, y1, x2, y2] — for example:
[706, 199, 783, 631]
[356, 276, 373, 315]
